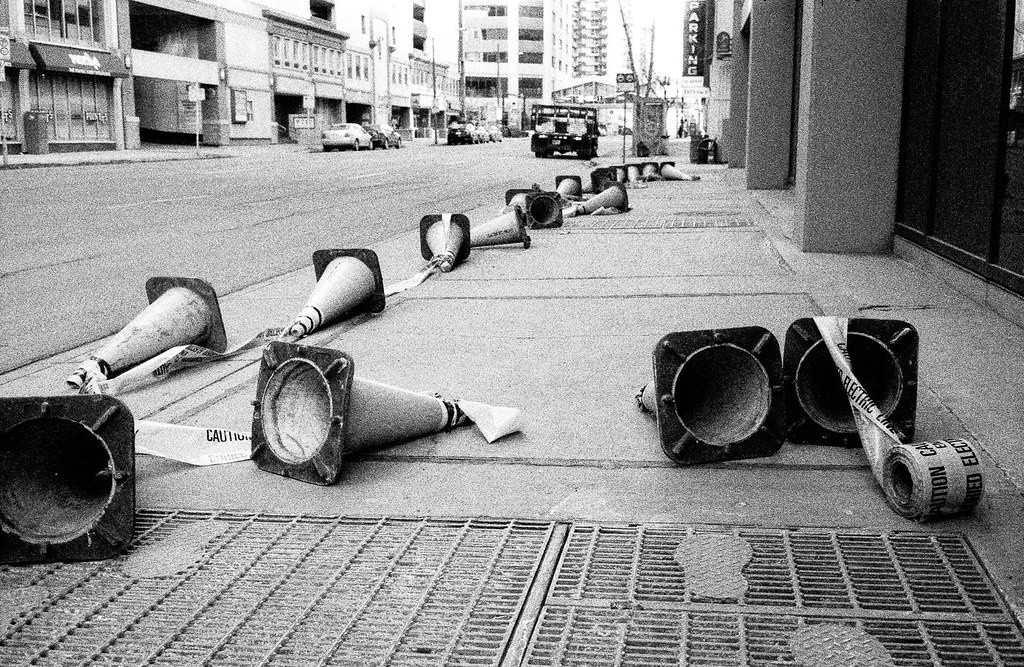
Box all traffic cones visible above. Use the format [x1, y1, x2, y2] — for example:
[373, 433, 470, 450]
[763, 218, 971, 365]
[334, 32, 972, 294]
[634, 323, 789, 466]
[589, 160, 696, 194]
[419, 212, 472, 272]
[573, 180, 630, 216]
[289, 248, 387, 337]
[555, 174, 583, 202]
[505, 183, 564, 230]
[0, 394, 140, 569]
[469, 203, 531, 250]
[59, 273, 230, 393]
[779, 314, 919, 450]
[249, 339, 474, 488]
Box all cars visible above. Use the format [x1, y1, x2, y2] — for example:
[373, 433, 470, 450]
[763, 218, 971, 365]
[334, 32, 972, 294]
[446, 124, 503, 145]
[321, 122, 374, 151]
[361, 123, 403, 149]
